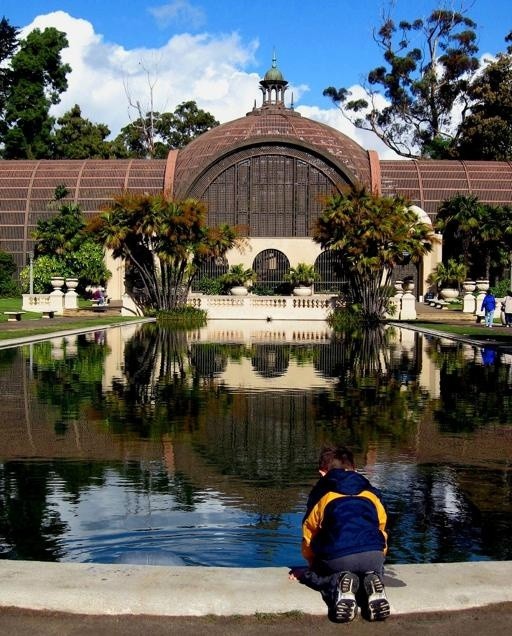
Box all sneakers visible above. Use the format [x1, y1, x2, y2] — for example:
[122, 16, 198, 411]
[331, 572, 360, 622]
[363, 572, 391, 622]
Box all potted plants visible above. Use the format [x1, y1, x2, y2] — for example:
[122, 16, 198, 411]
[283, 262, 322, 295]
[218, 262, 257, 296]
[425, 258, 469, 299]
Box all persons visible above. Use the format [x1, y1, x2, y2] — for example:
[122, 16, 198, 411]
[300, 442, 392, 623]
[500, 290, 512, 328]
[93, 288, 105, 305]
[480, 289, 497, 328]
